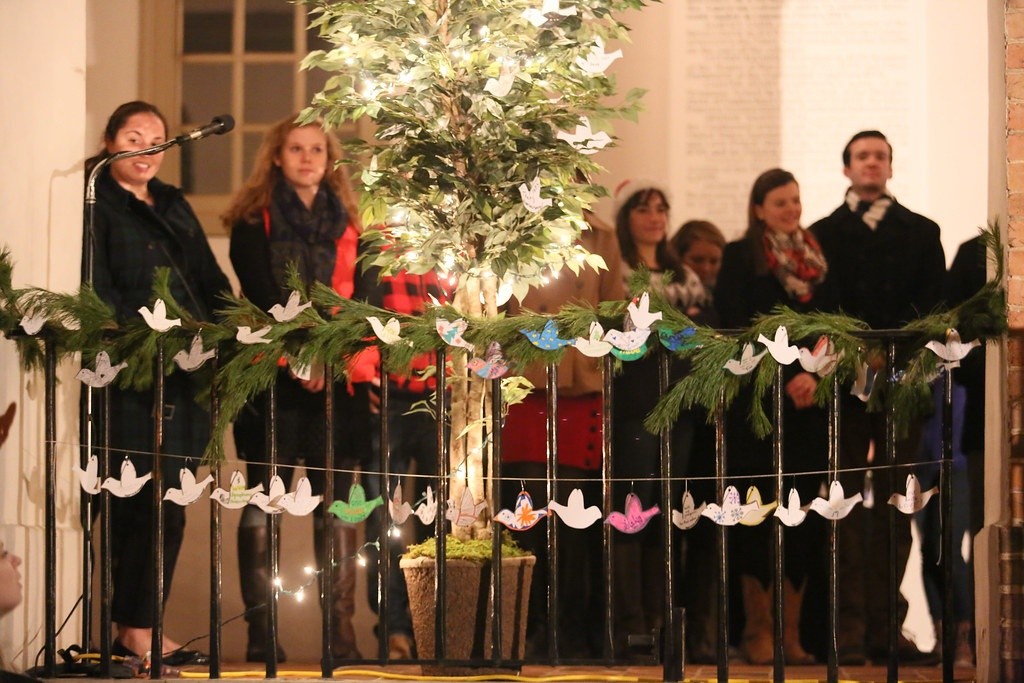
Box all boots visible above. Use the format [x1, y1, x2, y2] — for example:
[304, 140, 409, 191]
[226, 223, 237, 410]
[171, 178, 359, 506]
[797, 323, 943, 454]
[614, 542, 644, 652]
[783, 578, 815, 665]
[742, 576, 773, 663]
[312, 517, 363, 659]
[237, 523, 286, 661]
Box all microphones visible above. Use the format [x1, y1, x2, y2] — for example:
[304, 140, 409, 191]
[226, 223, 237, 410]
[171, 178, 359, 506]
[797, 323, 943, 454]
[176, 114, 235, 145]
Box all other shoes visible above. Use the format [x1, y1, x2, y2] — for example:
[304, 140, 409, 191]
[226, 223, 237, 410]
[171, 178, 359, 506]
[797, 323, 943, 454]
[876, 649, 939, 665]
[839, 647, 864, 665]
[112, 637, 199, 667]
[187, 649, 210, 666]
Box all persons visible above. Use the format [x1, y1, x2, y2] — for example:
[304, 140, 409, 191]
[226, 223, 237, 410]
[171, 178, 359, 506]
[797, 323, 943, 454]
[83, 100, 245, 665]
[808, 131, 951, 666]
[0, 534, 47, 683]
[504, 162, 625, 663]
[229, 112, 382, 665]
[334, 161, 464, 659]
[601, 183, 712, 663]
[721, 167, 842, 668]
[948, 216, 1001, 662]
[671, 219, 725, 293]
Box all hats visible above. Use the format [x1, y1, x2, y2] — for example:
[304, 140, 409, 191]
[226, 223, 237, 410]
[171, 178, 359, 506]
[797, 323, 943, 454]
[611, 179, 669, 220]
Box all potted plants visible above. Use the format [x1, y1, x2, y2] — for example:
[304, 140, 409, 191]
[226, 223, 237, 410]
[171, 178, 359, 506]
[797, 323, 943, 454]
[285, 0, 668, 677]
